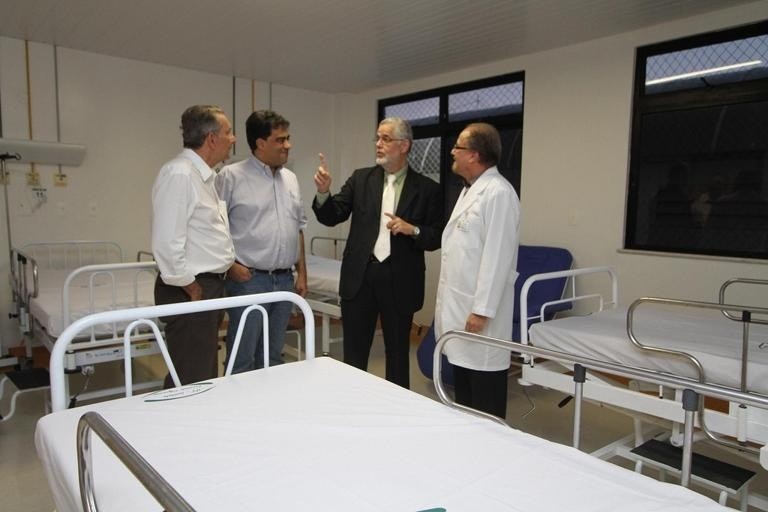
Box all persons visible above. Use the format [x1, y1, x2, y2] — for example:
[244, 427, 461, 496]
[310, 115, 442, 391]
[434, 122, 522, 420]
[213, 109, 308, 377]
[150, 104, 237, 392]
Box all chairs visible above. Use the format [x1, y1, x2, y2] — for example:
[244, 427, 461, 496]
[416, 245, 574, 385]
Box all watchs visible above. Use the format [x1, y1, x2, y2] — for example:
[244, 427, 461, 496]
[412, 225, 421, 235]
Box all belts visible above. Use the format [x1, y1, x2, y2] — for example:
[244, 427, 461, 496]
[249, 268, 293, 274]
[194, 270, 230, 281]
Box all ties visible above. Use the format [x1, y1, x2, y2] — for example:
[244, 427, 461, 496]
[372, 174, 397, 263]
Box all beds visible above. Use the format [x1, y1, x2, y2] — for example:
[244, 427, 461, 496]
[287, 235, 346, 356]
[516, 265, 768, 512]
[0, 239, 229, 425]
[34, 292, 739, 512]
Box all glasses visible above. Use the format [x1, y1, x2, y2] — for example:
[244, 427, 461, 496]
[373, 135, 403, 144]
